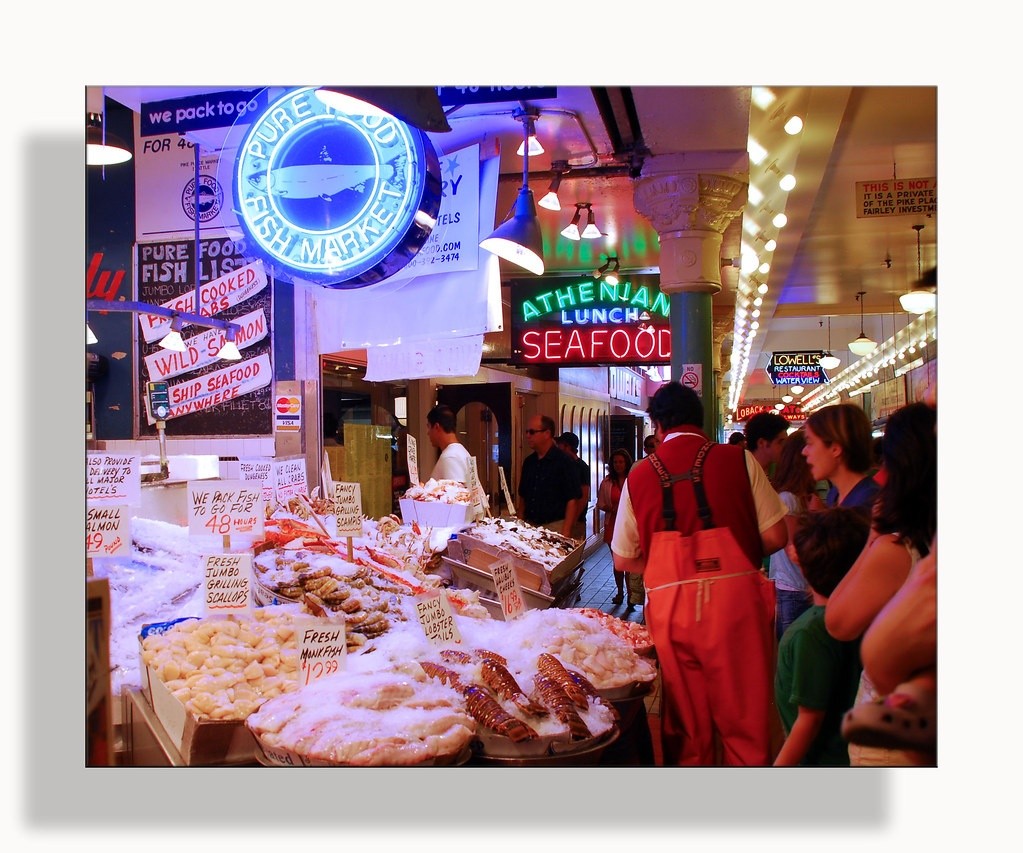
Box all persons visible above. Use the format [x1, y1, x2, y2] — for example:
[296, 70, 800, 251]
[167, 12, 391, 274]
[426, 405, 471, 489]
[596, 382, 937, 767]
[518, 415, 591, 541]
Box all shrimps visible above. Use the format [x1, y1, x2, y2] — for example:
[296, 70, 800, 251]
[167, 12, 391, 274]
[143, 482, 653, 762]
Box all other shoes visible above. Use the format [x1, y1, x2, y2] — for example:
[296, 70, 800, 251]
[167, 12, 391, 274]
[612, 595, 624, 601]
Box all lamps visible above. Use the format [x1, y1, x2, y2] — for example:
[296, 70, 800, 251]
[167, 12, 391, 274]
[86, 311, 244, 361]
[86, 122, 134, 166]
[768, 225, 937, 418]
[475, 104, 657, 334]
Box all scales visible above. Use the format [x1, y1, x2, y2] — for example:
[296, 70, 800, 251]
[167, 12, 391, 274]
[140, 381, 171, 481]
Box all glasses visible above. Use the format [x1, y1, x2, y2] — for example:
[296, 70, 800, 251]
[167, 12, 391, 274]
[526, 429, 544, 435]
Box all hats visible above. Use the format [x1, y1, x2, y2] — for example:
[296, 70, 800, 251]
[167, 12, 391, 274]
[553, 432, 579, 448]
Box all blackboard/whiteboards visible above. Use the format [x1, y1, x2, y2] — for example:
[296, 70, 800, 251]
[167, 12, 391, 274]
[133, 235, 274, 441]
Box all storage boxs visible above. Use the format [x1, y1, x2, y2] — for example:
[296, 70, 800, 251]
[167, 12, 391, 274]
[399, 493, 474, 529]
[135, 630, 263, 767]
